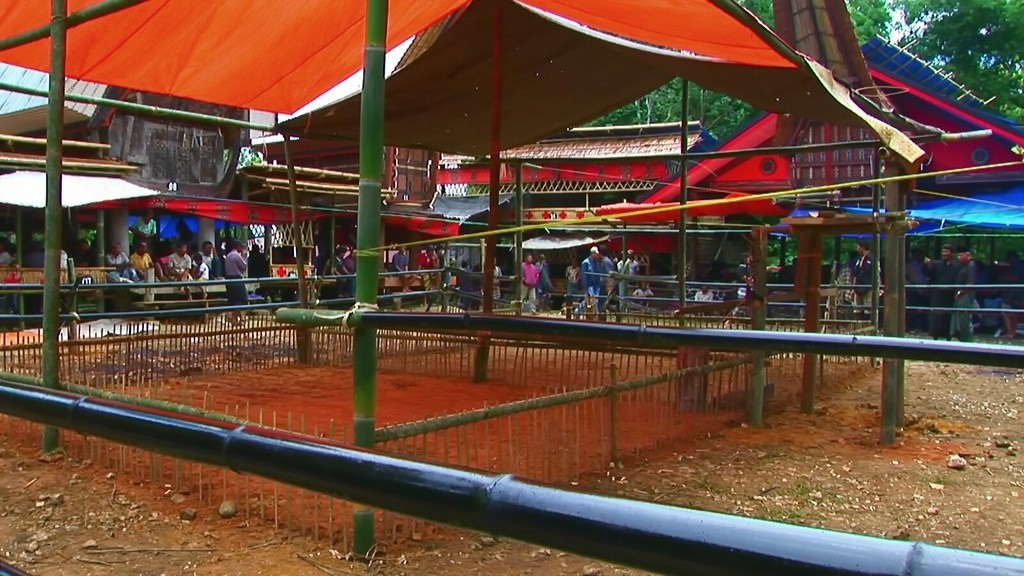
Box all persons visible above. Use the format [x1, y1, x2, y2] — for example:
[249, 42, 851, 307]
[0, 234, 1024, 342]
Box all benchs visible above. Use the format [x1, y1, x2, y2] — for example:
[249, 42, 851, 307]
[128, 295, 266, 324]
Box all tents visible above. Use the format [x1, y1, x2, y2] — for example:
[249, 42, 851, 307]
[0, 0, 949, 156]
